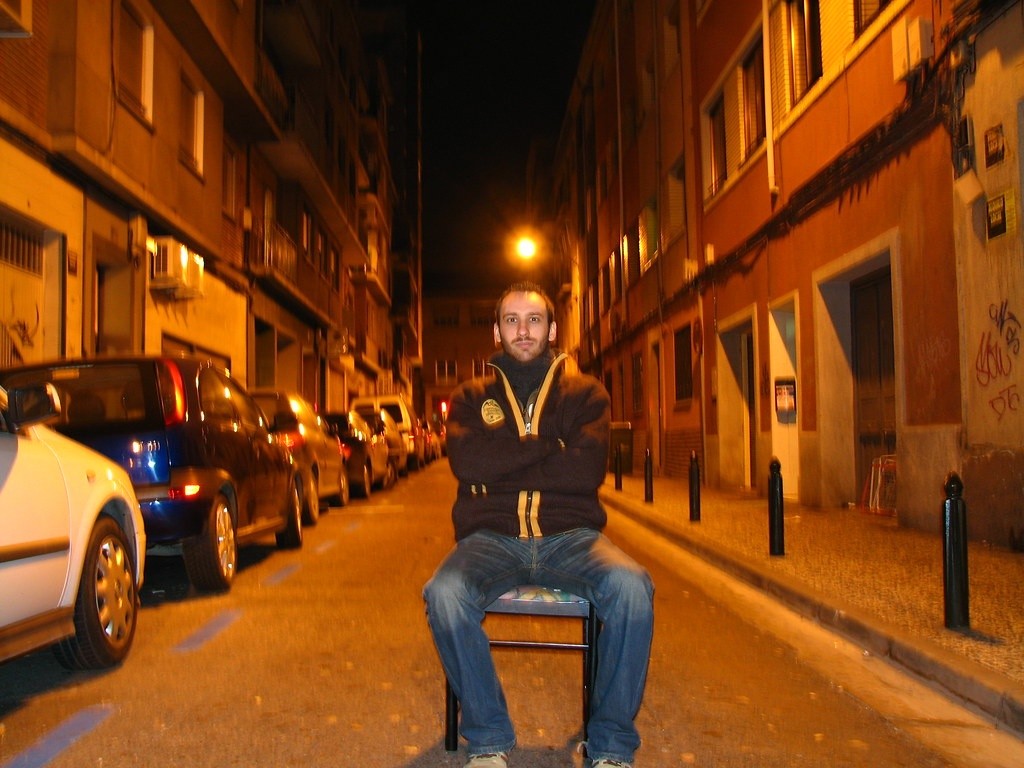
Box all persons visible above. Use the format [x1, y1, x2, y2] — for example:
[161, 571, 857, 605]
[421, 282, 655, 767]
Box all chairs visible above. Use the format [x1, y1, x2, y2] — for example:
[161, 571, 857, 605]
[67, 392, 105, 423]
[121, 381, 145, 419]
[445, 586, 601, 753]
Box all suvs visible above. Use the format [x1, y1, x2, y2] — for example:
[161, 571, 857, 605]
[0, 352, 310, 593]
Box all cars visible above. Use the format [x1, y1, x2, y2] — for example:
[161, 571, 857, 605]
[361, 407, 413, 477]
[243, 386, 351, 523]
[417, 415, 448, 464]
[322, 410, 395, 499]
[1, 372, 148, 673]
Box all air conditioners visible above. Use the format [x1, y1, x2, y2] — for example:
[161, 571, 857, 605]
[154, 237, 204, 299]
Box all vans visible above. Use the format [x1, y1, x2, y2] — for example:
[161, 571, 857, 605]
[351, 393, 424, 473]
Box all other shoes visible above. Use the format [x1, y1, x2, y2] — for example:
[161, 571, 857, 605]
[591, 756, 632, 768]
[462, 750, 510, 768]
